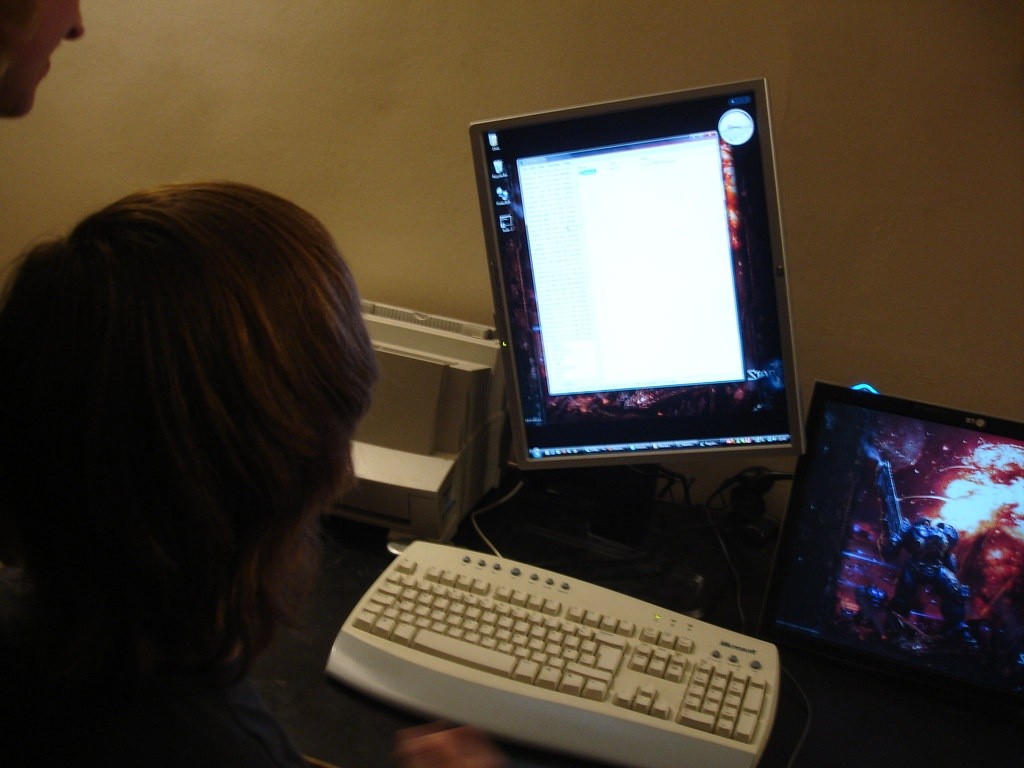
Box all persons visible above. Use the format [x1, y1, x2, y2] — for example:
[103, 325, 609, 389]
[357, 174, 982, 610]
[0, 0, 88, 119]
[0, 182, 504, 768]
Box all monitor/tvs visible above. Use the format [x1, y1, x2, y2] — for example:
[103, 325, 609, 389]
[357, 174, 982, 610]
[757, 380, 1024, 710]
[468, 74, 807, 619]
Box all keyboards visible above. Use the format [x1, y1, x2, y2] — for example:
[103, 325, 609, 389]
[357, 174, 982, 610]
[323, 540, 779, 768]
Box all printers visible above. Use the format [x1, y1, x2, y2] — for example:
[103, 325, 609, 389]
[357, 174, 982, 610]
[318, 298, 507, 541]
